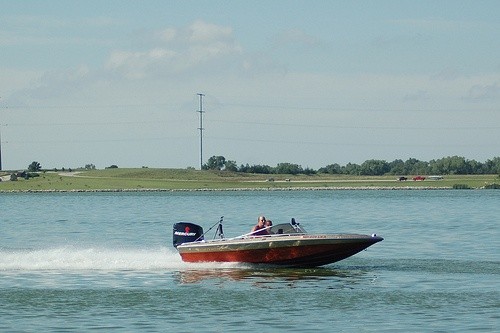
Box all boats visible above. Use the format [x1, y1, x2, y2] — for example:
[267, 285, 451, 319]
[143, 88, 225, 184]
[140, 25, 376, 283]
[171, 214, 384, 269]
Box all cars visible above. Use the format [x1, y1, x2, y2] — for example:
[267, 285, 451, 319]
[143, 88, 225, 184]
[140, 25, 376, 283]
[411, 175, 426, 181]
[264, 176, 275, 182]
[396, 176, 407, 181]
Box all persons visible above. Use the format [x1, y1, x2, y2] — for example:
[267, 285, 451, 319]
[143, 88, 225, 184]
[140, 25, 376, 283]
[250, 216, 271, 240]
[264, 220, 275, 235]
